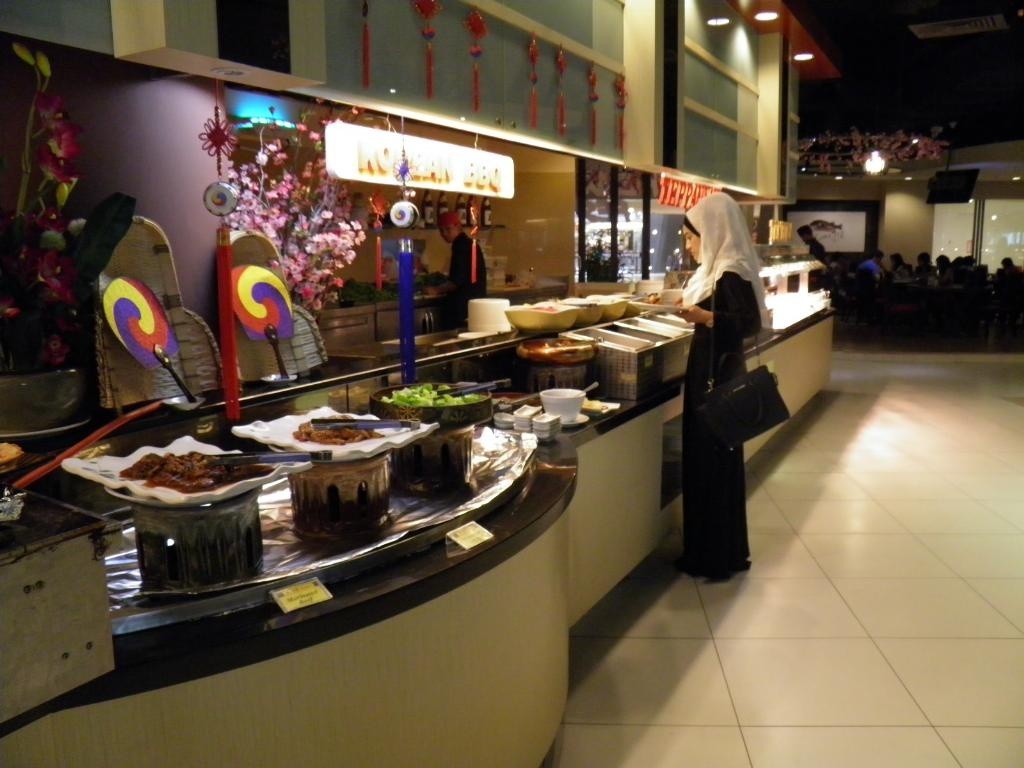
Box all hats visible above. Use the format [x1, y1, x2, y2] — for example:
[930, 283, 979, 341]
[437, 211, 462, 227]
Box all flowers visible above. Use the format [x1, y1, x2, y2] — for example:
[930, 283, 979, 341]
[801, 130, 947, 173]
[0, 41, 137, 378]
[224, 97, 394, 316]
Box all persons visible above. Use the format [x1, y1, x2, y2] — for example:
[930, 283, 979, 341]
[821, 244, 1024, 337]
[668, 192, 769, 581]
[437, 210, 488, 332]
[795, 224, 827, 293]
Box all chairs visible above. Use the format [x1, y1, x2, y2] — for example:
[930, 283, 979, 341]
[824, 256, 1024, 347]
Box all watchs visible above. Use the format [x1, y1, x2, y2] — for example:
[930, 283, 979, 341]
[705, 310, 715, 328]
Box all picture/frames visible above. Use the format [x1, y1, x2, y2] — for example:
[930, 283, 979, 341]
[781, 199, 880, 262]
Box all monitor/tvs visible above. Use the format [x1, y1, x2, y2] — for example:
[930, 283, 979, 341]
[926, 167, 980, 203]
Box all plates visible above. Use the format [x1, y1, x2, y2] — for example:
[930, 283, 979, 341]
[628, 295, 667, 312]
[502, 288, 633, 331]
[62, 432, 313, 505]
[494, 402, 563, 444]
[467, 298, 516, 335]
[560, 412, 591, 429]
[233, 405, 441, 462]
[582, 397, 621, 416]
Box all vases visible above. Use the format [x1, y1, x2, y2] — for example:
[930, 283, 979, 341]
[0, 368, 93, 433]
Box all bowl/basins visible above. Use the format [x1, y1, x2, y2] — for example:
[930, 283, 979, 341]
[541, 387, 586, 421]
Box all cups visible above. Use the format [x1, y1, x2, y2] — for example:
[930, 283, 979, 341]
[660, 288, 684, 314]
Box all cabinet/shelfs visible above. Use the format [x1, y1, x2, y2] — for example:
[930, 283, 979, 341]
[0, 260, 832, 768]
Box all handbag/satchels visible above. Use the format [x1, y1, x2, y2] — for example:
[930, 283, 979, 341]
[703, 364, 790, 445]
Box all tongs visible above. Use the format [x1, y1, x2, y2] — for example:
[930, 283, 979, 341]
[433, 377, 514, 402]
[311, 417, 422, 431]
[201, 448, 332, 468]
